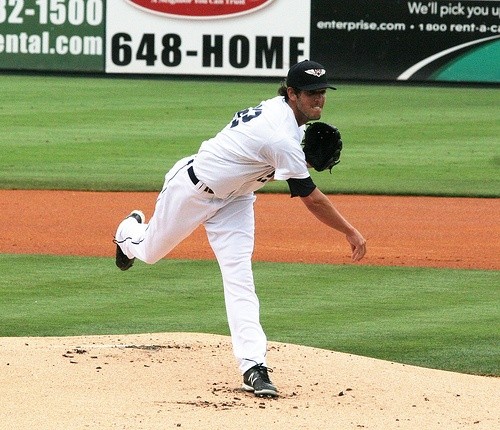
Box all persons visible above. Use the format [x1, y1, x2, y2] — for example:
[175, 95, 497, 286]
[114, 59, 365, 397]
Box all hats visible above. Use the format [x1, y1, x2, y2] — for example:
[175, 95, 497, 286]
[286, 58, 337, 91]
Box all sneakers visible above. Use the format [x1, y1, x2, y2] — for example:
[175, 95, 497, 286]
[243, 359, 280, 397]
[112, 210, 145, 271]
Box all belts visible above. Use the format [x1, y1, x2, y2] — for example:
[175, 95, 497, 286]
[186, 160, 214, 194]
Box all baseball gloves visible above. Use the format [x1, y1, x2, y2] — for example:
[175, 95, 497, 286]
[305, 122, 344, 173]
